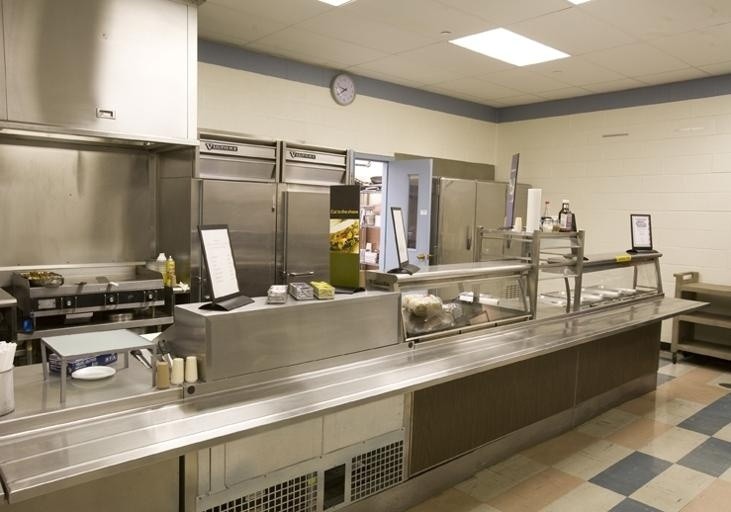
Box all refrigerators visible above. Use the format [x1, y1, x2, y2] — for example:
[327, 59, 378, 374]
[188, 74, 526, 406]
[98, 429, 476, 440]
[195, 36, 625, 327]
[410, 175, 533, 265]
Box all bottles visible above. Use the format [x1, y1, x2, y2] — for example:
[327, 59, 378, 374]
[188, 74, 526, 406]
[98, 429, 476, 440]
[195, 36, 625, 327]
[156, 251, 176, 288]
[558, 199, 573, 232]
[540, 200, 555, 234]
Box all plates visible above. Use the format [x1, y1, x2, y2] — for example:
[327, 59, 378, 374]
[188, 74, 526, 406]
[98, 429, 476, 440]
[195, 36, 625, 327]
[70, 365, 117, 381]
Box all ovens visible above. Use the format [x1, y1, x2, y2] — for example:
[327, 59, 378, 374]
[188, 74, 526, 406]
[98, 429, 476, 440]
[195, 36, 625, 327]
[156, 177, 350, 305]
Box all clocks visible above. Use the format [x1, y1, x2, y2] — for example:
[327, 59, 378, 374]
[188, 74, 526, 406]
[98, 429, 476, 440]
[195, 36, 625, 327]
[331, 74, 356, 106]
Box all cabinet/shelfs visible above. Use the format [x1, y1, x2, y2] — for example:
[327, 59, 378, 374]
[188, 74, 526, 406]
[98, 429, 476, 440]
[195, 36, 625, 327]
[366, 225, 663, 343]
[671, 272, 731, 367]
